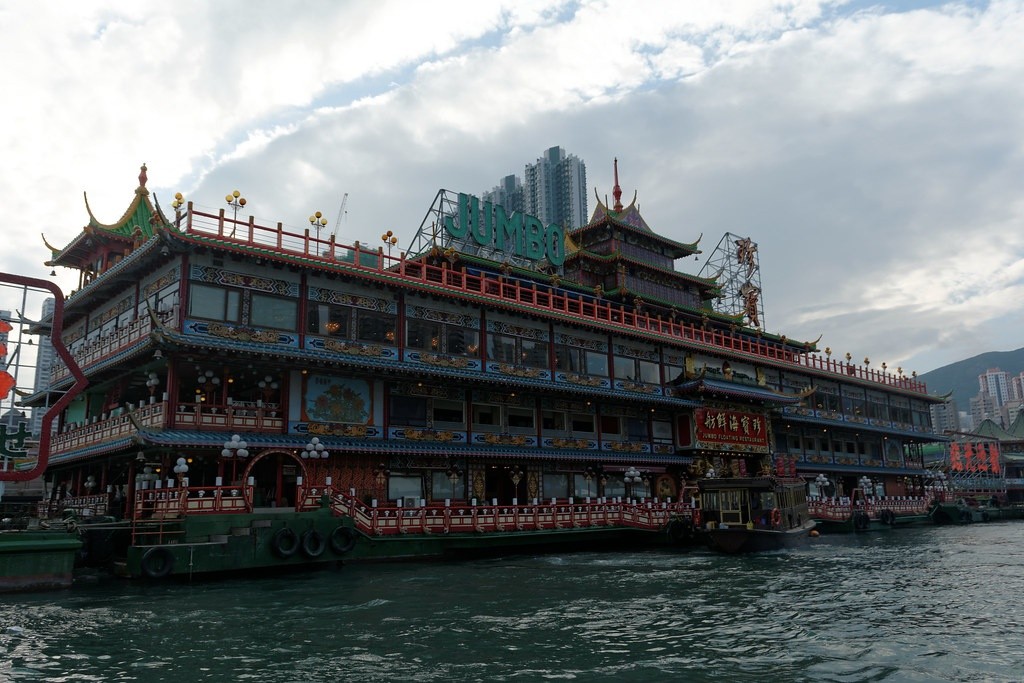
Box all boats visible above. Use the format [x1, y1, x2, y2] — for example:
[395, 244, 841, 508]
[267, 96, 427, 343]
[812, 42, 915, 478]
[666, 474, 817, 550]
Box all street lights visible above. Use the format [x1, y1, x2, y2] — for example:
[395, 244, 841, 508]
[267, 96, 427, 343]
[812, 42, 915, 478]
[131, 193, 185, 246]
[141, 466, 153, 490]
[921, 469, 947, 494]
[443, 245, 460, 285]
[624, 467, 652, 497]
[382, 230, 397, 271]
[446, 463, 463, 502]
[84, 475, 96, 494]
[680, 472, 688, 488]
[226, 190, 246, 239]
[583, 467, 610, 498]
[173, 457, 189, 488]
[309, 212, 327, 256]
[301, 437, 329, 485]
[258, 375, 279, 403]
[374, 463, 390, 501]
[498, 260, 918, 392]
[221, 434, 249, 485]
[858, 476, 879, 501]
[146, 372, 160, 395]
[815, 474, 844, 498]
[197, 370, 220, 404]
[509, 465, 523, 498]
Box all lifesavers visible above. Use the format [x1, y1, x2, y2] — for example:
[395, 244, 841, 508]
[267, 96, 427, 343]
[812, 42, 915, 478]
[771, 507, 781, 527]
[140, 546, 175, 577]
[273, 530, 300, 558]
[855, 514, 871, 529]
[301, 529, 327, 558]
[983, 511, 989, 521]
[882, 510, 896, 525]
[968, 512, 974, 521]
[330, 525, 357, 553]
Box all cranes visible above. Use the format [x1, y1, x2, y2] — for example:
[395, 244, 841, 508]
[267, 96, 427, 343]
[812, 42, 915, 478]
[331, 193, 349, 235]
